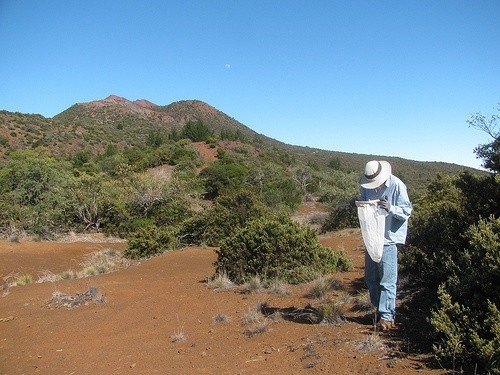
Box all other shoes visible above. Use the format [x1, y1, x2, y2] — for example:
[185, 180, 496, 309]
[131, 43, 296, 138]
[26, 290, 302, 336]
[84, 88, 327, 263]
[373, 320, 395, 332]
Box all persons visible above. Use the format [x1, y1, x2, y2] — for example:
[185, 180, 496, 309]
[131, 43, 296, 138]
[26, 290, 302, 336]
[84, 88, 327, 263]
[359, 160, 413, 328]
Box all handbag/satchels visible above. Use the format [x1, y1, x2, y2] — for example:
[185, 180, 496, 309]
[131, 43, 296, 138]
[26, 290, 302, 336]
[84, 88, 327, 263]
[355, 198, 386, 263]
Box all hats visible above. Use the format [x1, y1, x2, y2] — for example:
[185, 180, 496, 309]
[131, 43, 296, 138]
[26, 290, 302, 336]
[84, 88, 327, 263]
[359, 160, 391, 189]
